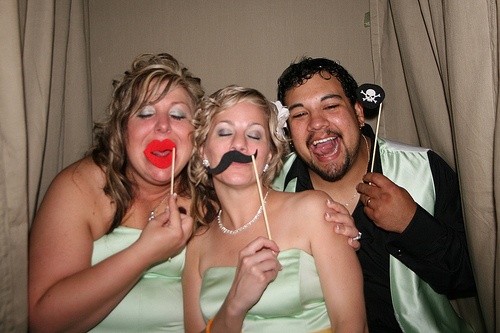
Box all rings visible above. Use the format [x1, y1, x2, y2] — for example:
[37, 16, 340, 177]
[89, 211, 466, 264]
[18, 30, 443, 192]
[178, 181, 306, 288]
[366, 195, 375, 207]
[352, 228, 362, 239]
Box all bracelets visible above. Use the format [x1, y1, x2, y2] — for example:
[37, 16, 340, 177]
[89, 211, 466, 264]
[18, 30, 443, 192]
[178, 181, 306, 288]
[205, 317, 215, 333]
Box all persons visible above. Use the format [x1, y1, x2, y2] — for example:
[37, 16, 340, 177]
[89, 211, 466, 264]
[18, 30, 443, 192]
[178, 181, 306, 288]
[181, 84, 370, 332]
[264, 56, 477, 332]
[26, 52, 223, 333]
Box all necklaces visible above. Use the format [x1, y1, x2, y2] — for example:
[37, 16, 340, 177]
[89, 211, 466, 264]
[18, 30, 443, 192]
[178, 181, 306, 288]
[307, 132, 372, 207]
[137, 182, 179, 223]
[217, 187, 271, 234]
